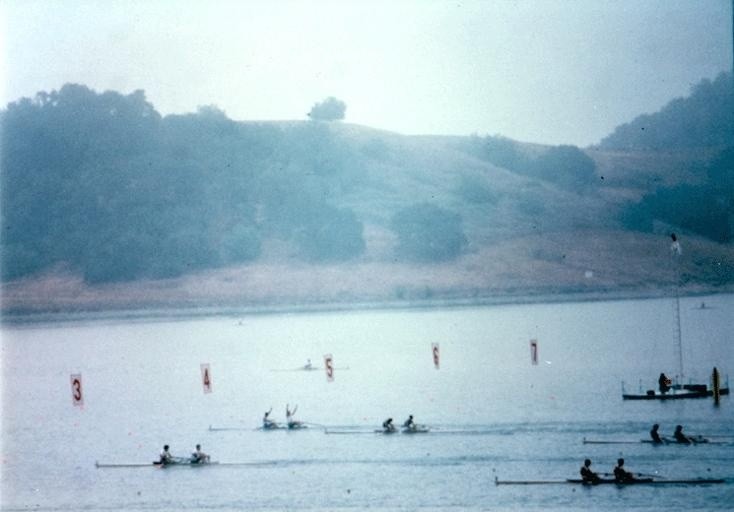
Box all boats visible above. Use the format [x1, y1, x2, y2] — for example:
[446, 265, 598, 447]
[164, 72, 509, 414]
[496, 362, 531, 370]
[90, 459, 307, 468]
[323, 424, 517, 435]
[208, 423, 327, 431]
[492, 476, 727, 484]
[582, 436, 709, 444]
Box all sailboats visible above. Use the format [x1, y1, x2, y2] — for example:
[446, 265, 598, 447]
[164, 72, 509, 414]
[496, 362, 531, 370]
[618, 232, 731, 399]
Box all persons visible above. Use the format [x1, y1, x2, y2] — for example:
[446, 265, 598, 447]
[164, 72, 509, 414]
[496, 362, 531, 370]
[405, 415, 416, 432]
[191, 444, 210, 465]
[657, 372, 671, 394]
[649, 423, 661, 441]
[262, 407, 278, 427]
[285, 403, 303, 427]
[382, 418, 395, 432]
[674, 424, 689, 441]
[579, 458, 599, 481]
[613, 458, 634, 480]
[158, 444, 172, 464]
